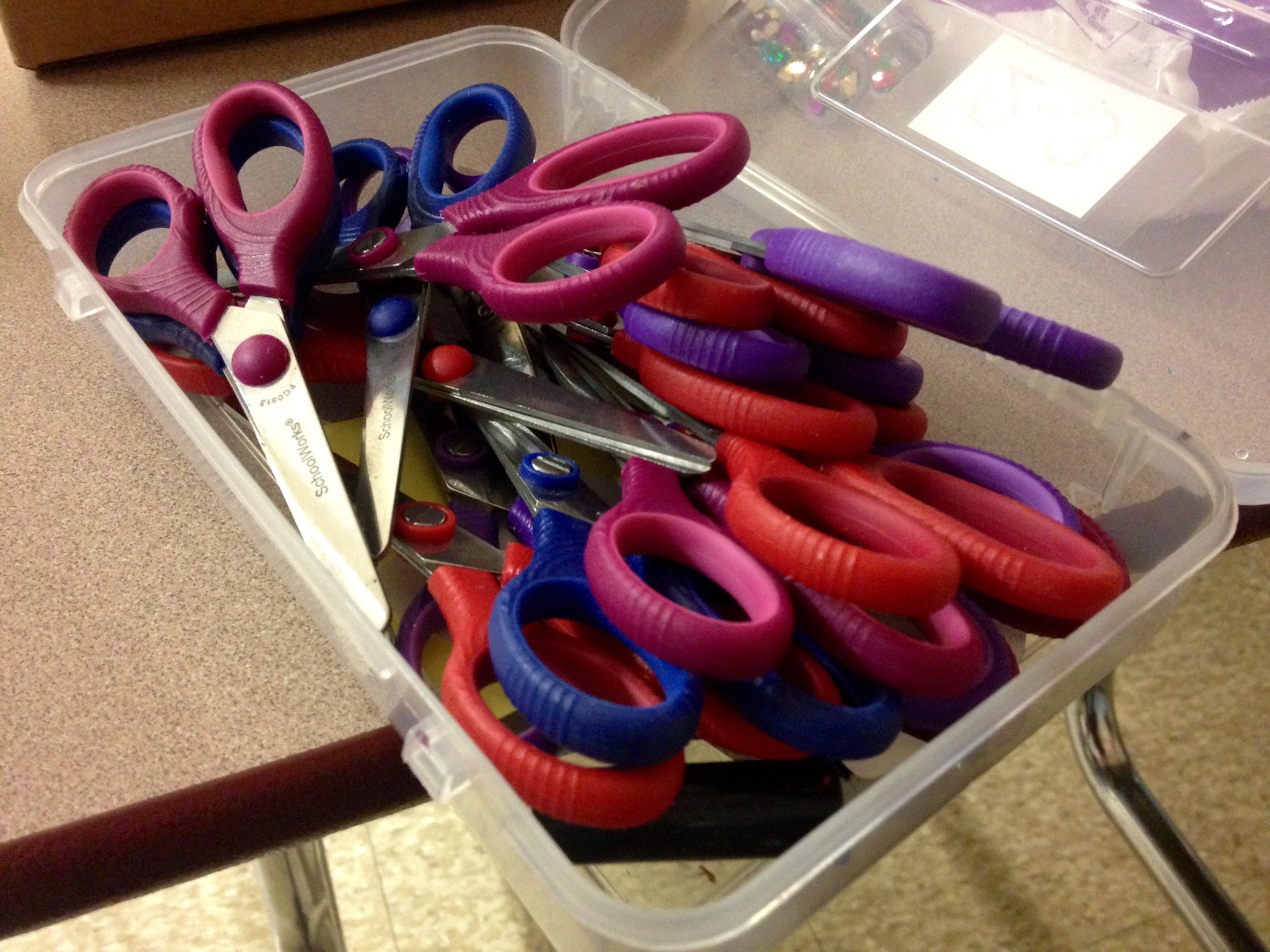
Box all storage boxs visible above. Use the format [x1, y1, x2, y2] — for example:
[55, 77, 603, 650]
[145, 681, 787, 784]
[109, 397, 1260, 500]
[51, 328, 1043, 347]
[12, 0, 1270, 950]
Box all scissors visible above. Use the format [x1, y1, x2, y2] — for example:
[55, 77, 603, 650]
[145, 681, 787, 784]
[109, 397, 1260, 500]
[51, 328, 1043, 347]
[58, 77, 1135, 867]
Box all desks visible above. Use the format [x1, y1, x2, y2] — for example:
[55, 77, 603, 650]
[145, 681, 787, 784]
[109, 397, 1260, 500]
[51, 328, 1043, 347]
[0, 0, 1270, 952]
